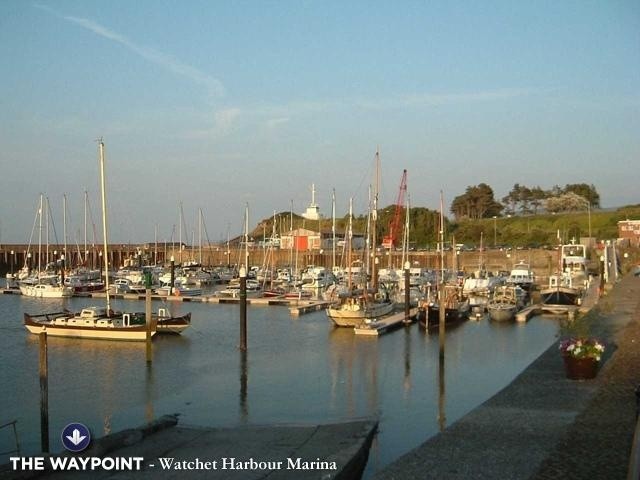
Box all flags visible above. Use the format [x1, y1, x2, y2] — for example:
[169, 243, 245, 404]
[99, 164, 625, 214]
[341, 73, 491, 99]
[279, 234, 321, 250]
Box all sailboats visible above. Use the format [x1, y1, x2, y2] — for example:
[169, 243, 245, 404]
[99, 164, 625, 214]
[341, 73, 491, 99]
[0, 133, 604, 345]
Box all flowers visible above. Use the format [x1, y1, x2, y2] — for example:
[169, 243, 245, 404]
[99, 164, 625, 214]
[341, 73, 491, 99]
[557, 337, 608, 361]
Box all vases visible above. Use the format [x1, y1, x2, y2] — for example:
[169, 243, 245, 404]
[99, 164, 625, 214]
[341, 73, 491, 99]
[563, 355, 599, 378]
